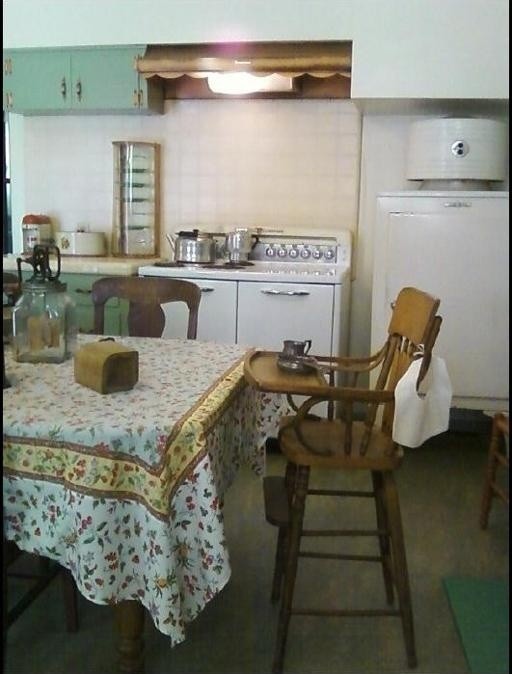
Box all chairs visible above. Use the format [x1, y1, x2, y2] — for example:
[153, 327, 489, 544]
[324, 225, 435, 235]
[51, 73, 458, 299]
[478, 414, 509, 528]
[244, 287, 443, 673]
[88, 276, 201, 339]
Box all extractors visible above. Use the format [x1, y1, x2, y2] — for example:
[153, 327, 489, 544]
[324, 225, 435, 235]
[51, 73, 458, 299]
[138, 40, 352, 99]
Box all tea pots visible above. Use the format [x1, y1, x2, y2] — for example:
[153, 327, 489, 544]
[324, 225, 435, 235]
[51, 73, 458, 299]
[166, 228, 217, 262]
[224, 232, 259, 264]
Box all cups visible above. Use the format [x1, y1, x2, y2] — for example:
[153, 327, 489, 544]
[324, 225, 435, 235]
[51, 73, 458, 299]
[283, 339, 311, 361]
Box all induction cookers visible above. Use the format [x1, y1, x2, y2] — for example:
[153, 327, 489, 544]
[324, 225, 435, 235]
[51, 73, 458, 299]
[137, 255, 255, 282]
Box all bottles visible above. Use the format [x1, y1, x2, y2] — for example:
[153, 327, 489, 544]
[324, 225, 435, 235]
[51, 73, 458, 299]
[11, 281, 78, 363]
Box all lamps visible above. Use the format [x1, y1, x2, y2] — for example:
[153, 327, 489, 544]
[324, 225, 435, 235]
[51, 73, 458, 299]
[207, 69, 299, 95]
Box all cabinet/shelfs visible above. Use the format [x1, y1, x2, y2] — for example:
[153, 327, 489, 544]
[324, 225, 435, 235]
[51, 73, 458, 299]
[3, 45, 167, 114]
[1, 258, 144, 335]
[136, 268, 337, 443]
[366, 190, 509, 415]
